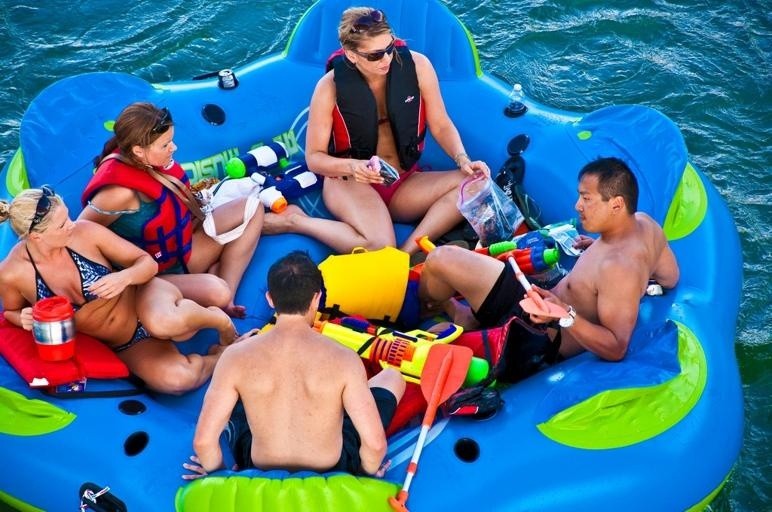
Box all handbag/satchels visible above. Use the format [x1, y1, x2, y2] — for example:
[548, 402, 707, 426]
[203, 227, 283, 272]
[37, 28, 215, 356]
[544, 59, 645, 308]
[196, 175, 261, 246]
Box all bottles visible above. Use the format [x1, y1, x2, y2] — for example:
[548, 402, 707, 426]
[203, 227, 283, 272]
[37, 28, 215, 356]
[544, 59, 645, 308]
[507, 84, 526, 112]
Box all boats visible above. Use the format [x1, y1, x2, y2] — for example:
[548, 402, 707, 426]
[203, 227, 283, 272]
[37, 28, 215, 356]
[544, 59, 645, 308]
[0, 0, 745, 512]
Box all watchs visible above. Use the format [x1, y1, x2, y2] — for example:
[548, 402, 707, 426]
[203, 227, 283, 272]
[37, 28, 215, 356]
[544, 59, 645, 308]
[558, 302, 577, 329]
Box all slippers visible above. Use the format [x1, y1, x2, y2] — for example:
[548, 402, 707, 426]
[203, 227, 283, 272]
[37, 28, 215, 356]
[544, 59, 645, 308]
[514, 184, 542, 231]
[492, 155, 525, 201]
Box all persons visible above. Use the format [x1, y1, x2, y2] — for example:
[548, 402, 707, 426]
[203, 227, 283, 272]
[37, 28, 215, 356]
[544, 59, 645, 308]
[75, 102, 267, 318]
[259, 7, 492, 258]
[416, 155, 680, 383]
[179, 249, 408, 476]
[0, 183, 260, 399]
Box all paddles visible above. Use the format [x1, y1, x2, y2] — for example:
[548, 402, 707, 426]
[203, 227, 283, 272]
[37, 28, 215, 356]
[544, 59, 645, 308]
[395, 342, 473, 509]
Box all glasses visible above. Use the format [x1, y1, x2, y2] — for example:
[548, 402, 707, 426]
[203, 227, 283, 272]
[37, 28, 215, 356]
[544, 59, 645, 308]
[148, 106, 174, 134]
[350, 34, 395, 63]
[28, 185, 56, 234]
